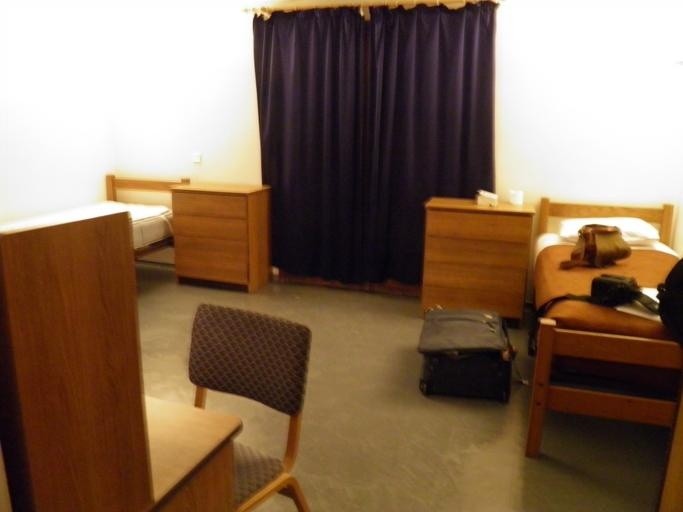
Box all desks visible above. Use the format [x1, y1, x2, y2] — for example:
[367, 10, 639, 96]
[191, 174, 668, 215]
[0, 211, 244, 512]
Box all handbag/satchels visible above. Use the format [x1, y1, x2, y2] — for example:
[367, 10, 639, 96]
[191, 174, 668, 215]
[559, 223, 633, 271]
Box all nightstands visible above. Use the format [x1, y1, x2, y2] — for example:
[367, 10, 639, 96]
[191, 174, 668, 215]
[421, 196, 537, 328]
[168, 184, 273, 293]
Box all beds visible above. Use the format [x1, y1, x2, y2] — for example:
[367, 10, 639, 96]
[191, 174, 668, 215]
[525, 198, 682, 458]
[0, 174, 191, 262]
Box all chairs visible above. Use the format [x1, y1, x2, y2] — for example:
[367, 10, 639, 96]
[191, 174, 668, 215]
[189, 303, 312, 512]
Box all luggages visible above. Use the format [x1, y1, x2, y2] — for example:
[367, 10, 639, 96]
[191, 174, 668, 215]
[418, 305, 515, 403]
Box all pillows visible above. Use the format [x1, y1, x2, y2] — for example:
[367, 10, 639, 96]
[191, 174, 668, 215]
[559, 216, 660, 247]
